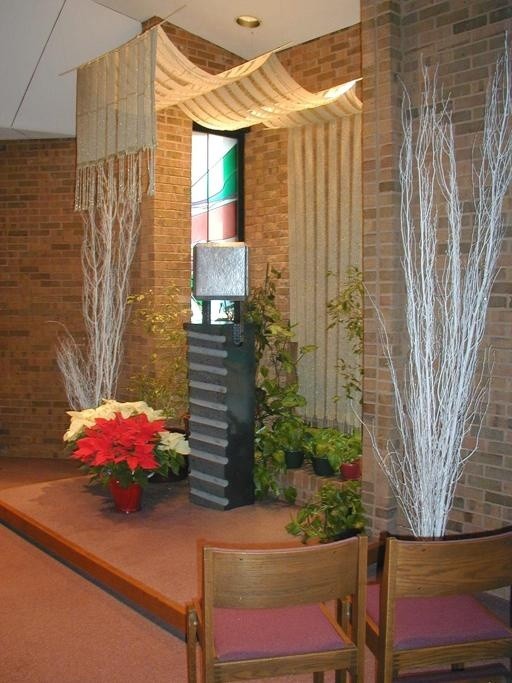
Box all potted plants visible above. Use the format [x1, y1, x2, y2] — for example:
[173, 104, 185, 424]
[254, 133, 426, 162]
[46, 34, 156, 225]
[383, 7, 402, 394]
[284, 479, 367, 546]
[271, 413, 363, 481]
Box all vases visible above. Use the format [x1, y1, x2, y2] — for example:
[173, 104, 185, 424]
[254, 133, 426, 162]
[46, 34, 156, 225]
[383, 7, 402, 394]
[108, 476, 143, 514]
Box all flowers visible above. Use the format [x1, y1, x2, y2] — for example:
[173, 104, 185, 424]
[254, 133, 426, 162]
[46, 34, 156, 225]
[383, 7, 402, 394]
[61, 399, 191, 491]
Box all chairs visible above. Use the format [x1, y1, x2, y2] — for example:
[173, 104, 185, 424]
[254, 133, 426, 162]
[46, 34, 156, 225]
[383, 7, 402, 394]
[183, 533, 368, 683]
[345, 526, 512, 683]
